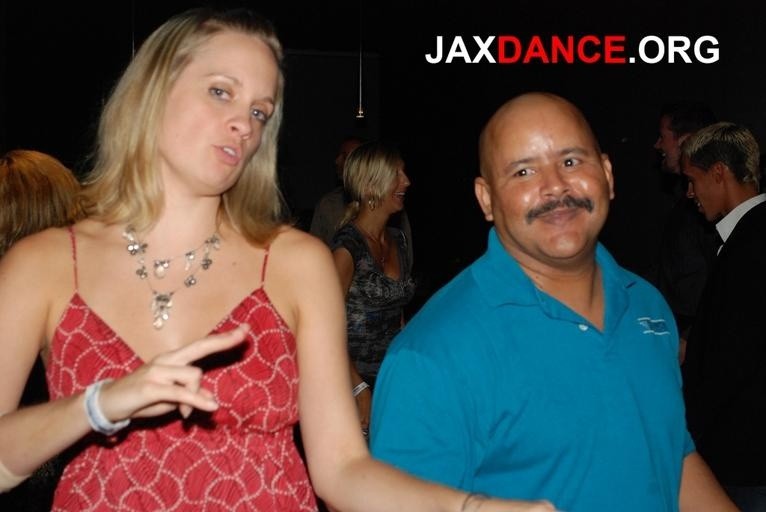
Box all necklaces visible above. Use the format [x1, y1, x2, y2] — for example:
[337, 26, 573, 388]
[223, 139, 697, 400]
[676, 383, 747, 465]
[122, 225, 221, 330]
[364, 226, 393, 267]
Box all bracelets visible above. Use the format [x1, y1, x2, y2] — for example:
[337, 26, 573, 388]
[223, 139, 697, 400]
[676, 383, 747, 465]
[84, 376, 131, 436]
[353, 381, 370, 397]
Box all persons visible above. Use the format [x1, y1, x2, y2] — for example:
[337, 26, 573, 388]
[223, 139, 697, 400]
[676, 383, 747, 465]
[2, 150, 82, 511]
[656, 93, 720, 329]
[370, 88, 742, 511]
[678, 122, 766, 511]
[1, 0, 559, 512]
[330, 138, 418, 426]
[310, 128, 417, 274]
